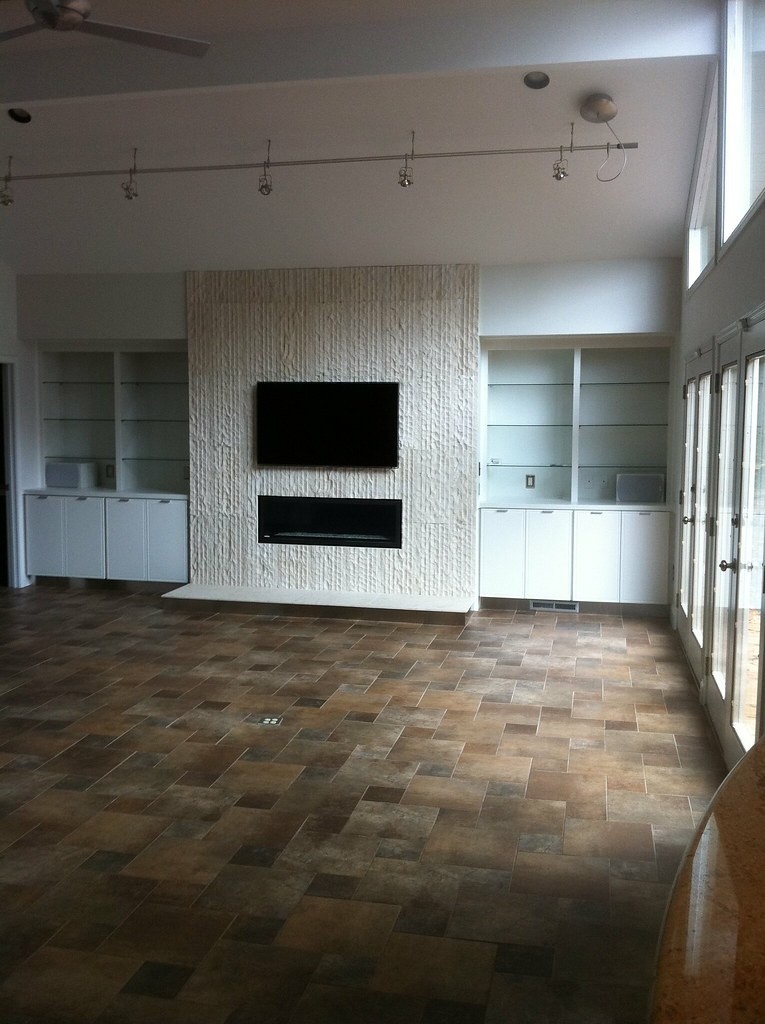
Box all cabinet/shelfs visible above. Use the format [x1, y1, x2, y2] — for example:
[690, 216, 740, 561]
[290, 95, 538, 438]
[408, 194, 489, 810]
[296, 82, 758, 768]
[577, 379, 670, 469]
[122, 380, 189, 461]
[42, 380, 115, 461]
[479, 508, 573, 601]
[572, 509, 675, 605]
[486, 382, 573, 469]
[105, 497, 190, 583]
[24, 494, 107, 579]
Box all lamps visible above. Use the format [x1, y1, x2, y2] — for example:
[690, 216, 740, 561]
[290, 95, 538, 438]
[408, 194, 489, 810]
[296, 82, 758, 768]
[258, 167, 273, 196]
[120, 174, 138, 200]
[0, 181, 15, 207]
[398, 157, 414, 187]
[552, 150, 569, 181]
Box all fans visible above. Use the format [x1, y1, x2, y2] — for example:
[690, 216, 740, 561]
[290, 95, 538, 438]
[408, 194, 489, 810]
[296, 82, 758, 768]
[0, 0, 211, 58]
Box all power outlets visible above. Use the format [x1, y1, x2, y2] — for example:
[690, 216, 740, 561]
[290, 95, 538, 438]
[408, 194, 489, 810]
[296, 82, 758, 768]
[600, 474, 609, 489]
[184, 465, 190, 479]
[106, 464, 114, 478]
[584, 475, 594, 489]
[525, 474, 535, 488]
